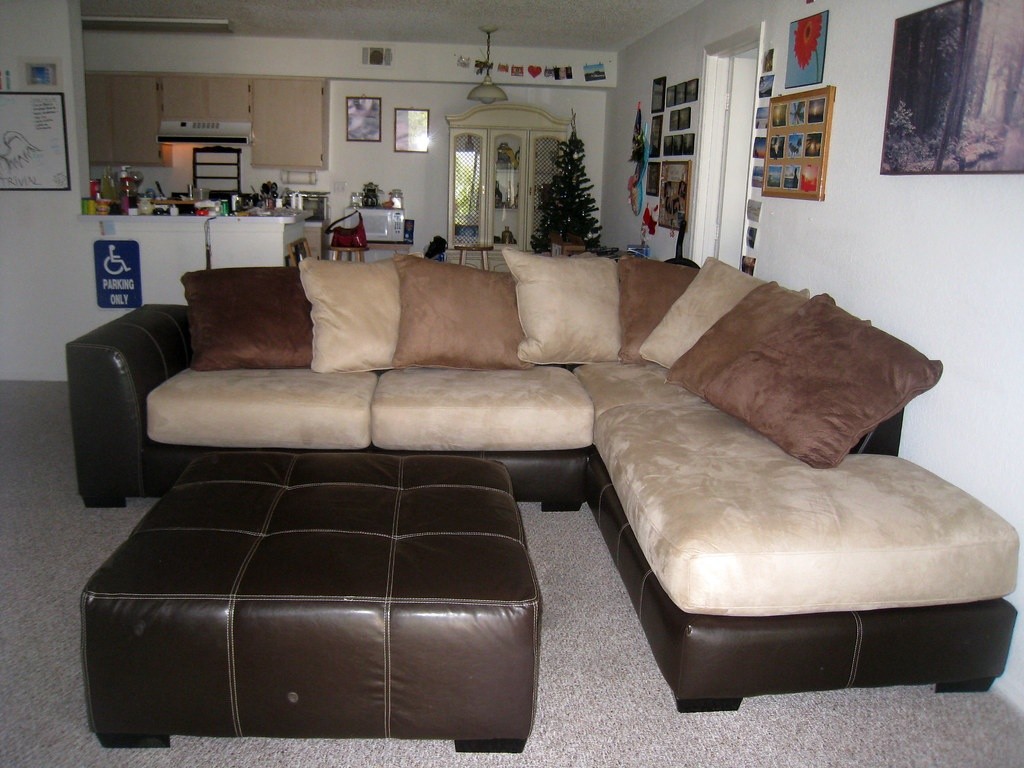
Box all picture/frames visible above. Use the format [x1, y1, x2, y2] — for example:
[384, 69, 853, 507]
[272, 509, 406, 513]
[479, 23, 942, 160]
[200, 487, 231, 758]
[394, 107, 430, 153]
[656, 158, 692, 230]
[651, 75, 667, 113]
[346, 95, 382, 142]
[647, 162, 661, 197]
[760, 85, 836, 202]
[649, 114, 663, 157]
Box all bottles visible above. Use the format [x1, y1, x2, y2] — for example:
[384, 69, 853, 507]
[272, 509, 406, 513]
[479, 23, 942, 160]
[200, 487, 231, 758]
[362, 188, 378, 207]
[119, 176, 138, 198]
[275, 198, 283, 207]
[350, 192, 363, 207]
[382, 201, 393, 208]
[220, 200, 229, 216]
[502, 226, 512, 243]
[138, 198, 152, 215]
[391, 191, 403, 208]
[101, 175, 117, 200]
[95, 199, 111, 216]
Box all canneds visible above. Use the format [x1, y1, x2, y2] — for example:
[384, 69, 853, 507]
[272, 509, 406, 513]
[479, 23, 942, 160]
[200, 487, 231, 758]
[220, 200, 229, 216]
[81, 199, 111, 216]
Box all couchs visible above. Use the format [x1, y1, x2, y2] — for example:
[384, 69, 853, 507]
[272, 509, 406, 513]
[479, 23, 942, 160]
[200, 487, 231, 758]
[64, 304, 594, 513]
[573, 295, 987, 714]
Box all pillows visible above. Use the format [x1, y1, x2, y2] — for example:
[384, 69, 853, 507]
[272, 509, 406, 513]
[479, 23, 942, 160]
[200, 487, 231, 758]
[181, 268, 320, 373]
[390, 253, 532, 369]
[704, 294, 944, 470]
[637, 258, 768, 370]
[499, 246, 626, 363]
[297, 255, 401, 374]
[617, 252, 700, 364]
[668, 281, 812, 401]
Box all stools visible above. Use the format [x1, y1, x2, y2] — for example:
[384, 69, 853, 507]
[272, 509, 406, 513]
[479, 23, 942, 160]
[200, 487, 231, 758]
[79, 454, 542, 753]
[325, 245, 369, 264]
[454, 244, 494, 273]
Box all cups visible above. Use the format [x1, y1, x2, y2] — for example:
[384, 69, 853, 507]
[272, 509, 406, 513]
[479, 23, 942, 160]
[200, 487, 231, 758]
[169, 207, 179, 216]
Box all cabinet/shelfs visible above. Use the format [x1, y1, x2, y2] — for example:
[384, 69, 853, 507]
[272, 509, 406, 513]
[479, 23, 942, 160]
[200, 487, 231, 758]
[442, 103, 578, 272]
[85, 72, 328, 168]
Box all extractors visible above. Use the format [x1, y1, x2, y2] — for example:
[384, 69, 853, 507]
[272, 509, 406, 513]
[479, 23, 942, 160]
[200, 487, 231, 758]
[155, 121, 253, 146]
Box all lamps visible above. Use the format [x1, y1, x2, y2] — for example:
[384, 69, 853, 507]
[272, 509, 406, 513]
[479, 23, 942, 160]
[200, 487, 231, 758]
[467, 24, 508, 104]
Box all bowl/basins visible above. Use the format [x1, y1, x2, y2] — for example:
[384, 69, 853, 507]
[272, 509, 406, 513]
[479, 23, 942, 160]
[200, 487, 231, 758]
[193, 188, 209, 200]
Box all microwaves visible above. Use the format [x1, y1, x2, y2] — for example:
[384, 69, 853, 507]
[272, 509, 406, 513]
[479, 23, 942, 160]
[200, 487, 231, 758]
[344, 206, 405, 243]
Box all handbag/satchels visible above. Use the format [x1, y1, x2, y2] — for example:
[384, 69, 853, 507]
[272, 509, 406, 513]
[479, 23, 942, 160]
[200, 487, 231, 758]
[324, 209, 368, 247]
